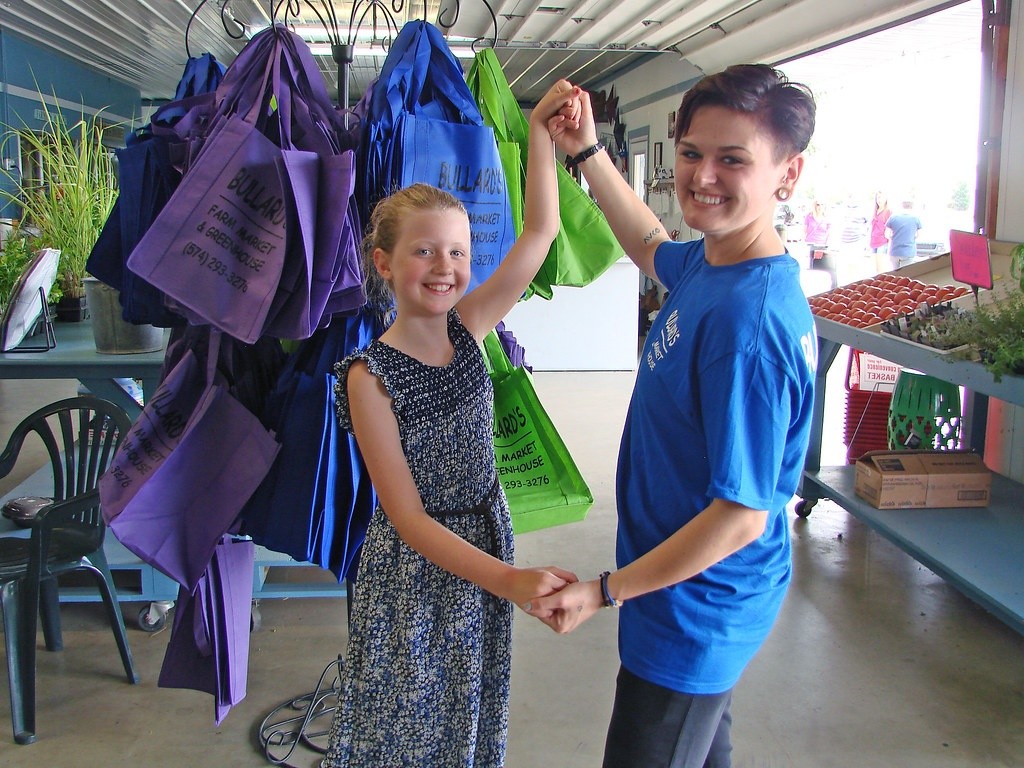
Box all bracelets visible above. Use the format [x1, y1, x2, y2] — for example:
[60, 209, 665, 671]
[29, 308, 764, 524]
[565, 141, 603, 167]
[599, 571, 622, 610]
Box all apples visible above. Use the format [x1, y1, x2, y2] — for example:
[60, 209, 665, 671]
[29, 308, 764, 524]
[805, 274, 973, 330]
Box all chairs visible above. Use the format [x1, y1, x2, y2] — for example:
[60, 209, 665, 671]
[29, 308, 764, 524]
[0, 398, 131, 746]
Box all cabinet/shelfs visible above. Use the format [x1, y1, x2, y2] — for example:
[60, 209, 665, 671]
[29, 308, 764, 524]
[796, 241, 1024, 639]
[0, 319, 348, 634]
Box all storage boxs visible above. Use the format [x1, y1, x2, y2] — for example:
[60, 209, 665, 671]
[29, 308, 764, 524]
[854, 450, 992, 510]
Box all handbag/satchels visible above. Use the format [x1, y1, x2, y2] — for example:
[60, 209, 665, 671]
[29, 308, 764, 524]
[85, 19, 628, 727]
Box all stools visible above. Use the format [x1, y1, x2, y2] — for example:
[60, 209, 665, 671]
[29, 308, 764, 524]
[887, 368, 962, 451]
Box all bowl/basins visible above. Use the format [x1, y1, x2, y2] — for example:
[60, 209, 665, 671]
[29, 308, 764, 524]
[0, 497, 53, 527]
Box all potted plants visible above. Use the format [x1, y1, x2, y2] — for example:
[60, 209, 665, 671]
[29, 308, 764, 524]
[0, 229, 63, 345]
[0, 61, 120, 322]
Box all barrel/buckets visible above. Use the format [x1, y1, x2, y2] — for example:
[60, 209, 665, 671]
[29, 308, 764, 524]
[83, 276, 164, 355]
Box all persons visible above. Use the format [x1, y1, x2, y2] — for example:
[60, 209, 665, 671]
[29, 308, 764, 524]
[523, 62, 821, 768]
[775, 190, 923, 290]
[324, 77, 582, 768]
